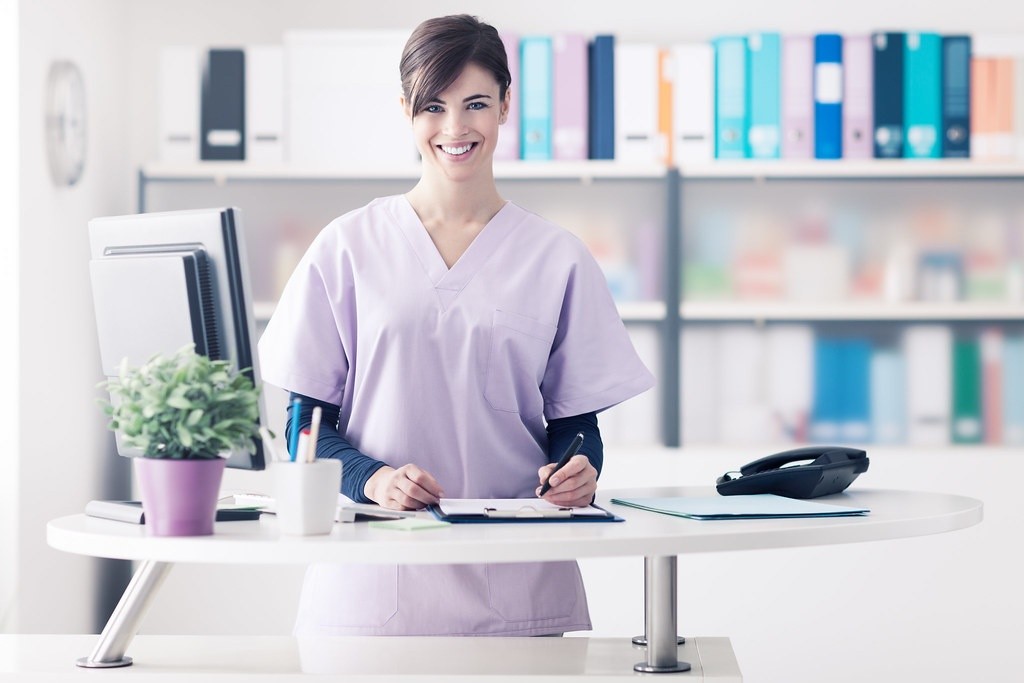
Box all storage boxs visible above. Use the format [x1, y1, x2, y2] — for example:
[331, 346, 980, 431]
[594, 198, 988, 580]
[162, 31, 1016, 159]
[775, 322, 1024, 449]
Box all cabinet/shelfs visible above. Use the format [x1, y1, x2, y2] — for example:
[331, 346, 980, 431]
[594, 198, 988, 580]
[136, 160, 1024, 449]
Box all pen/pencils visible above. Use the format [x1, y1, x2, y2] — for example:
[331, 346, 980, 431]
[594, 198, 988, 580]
[538, 431, 584, 500]
[259, 398, 322, 463]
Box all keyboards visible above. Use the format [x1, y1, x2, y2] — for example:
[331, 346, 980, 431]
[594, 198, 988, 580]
[220, 489, 416, 524]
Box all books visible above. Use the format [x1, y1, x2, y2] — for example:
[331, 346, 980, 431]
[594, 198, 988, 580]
[497, 32, 1024, 164]
[682, 324, 1024, 446]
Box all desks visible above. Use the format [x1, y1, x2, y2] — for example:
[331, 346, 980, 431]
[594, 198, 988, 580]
[0, 489, 984, 683]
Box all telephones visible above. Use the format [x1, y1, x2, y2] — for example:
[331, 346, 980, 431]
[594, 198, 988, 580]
[715, 445, 870, 498]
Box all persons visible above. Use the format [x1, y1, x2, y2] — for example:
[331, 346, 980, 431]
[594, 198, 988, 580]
[255, 14, 656, 638]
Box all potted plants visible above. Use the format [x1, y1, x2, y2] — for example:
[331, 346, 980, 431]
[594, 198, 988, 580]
[94, 342, 275, 537]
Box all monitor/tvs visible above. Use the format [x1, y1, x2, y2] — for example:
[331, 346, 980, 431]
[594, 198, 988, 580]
[86, 206, 275, 525]
